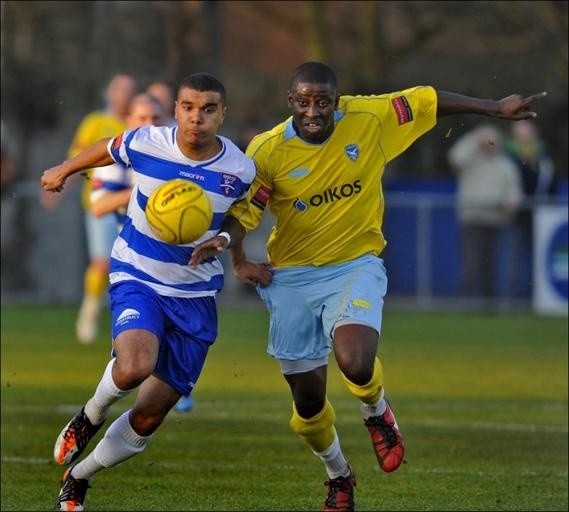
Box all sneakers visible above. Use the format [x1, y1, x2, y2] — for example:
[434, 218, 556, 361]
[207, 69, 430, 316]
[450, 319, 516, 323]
[322, 463, 356, 512]
[174, 393, 193, 412]
[364, 396, 404, 472]
[59, 460, 88, 512]
[54, 406, 106, 466]
[77, 297, 101, 343]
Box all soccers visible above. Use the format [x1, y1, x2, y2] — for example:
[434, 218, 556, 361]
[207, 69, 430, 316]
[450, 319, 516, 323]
[145, 178, 213, 244]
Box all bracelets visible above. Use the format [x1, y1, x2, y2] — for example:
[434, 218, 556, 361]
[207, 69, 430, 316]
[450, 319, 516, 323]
[217, 230, 231, 247]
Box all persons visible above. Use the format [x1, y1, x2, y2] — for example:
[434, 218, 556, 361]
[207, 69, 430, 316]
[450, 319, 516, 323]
[187, 62, 548, 512]
[65, 73, 175, 343]
[41, 73, 274, 511]
[444, 114, 558, 305]
